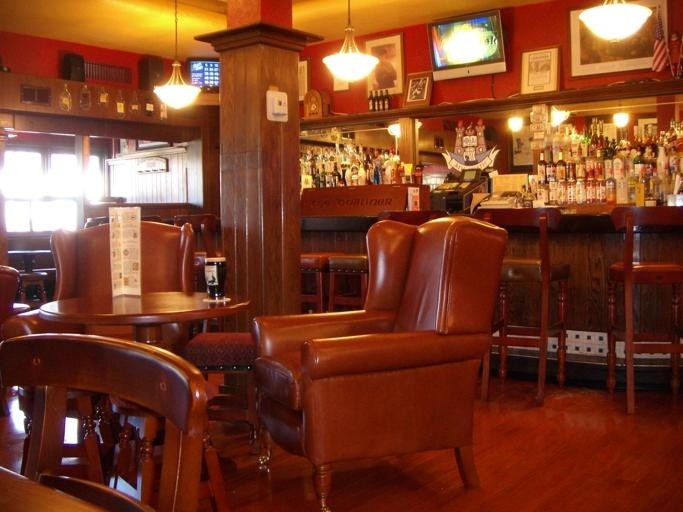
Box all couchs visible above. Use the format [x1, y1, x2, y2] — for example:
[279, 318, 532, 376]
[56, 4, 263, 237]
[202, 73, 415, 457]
[0, 264, 30, 316]
[249, 218, 507, 511]
[174, 213, 222, 259]
[0, 236, 56, 279]
[48, 220, 196, 444]
[85, 216, 162, 227]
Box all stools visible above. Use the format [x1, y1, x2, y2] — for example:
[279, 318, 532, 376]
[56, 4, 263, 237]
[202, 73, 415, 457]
[19, 272, 49, 305]
[190, 331, 258, 450]
[302, 252, 345, 312]
[328, 255, 368, 311]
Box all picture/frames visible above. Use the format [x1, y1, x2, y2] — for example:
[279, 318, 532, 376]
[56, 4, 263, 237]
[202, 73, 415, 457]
[403, 71, 433, 108]
[570, 0, 672, 77]
[364, 33, 405, 97]
[297, 56, 311, 102]
[519, 44, 559, 95]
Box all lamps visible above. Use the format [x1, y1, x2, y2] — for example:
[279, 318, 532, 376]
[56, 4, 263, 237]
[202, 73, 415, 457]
[551, 107, 569, 128]
[508, 116, 523, 133]
[322, 1, 379, 82]
[614, 112, 629, 129]
[579, 0, 652, 42]
[388, 123, 401, 137]
[152, 1, 201, 109]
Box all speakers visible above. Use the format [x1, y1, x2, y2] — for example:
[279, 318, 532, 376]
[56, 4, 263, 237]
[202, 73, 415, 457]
[138, 55, 163, 90]
[63, 54, 85, 81]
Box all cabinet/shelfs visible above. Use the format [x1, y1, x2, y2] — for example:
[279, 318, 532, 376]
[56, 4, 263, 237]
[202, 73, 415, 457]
[303, 183, 681, 394]
[82, 195, 202, 222]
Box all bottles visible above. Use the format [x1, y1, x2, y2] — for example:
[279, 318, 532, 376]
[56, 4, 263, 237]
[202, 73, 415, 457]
[520, 185, 526, 207]
[549, 178, 557, 203]
[568, 147, 575, 182]
[570, 128, 580, 159]
[407, 187, 420, 211]
[59, 84, 72, 113]
[558, 179, 567, 203]
[604, 149, 613, 178]
[556, 146, 566, 182]
[80, 84, 92, 111]
[584, 145, 595, 182]
[614, 155, 624, 176]
[562, 127, 570, 161]
[300, 151, 340, 188]
[543, 125, 551, 163]
[606, 168, 616, 203]
[547, 148, 557, 183]
[129, 90, 141, 116]
[384, 89, 389, 109]
[590, 118, 631, 155]
[526, 185, 533, 208]
[586, 178, 596, 204]
[552, 126, 560, 162]
[596, 178, 606, 204]
[631, 121, 683, 206]
[632, 226, 640, 265]
[536, 178, 547, 204]
[579, 129, 588, 144]
[346, 144, 423, 185]
[577, 179, 586, 203]
[568, 178, 577, 203]
[576, 145, 586, 180]
[159, 101, 168, 120]
[596, 149, 606, 180]
[144, 94, 154, 116]
[114, 89, 126, 119]
[537, 150, 547, 184]
[617, 167, 629, 204]
[97, 86, 110, 109]
[379, 89, 384, 110]
[374, 90, 379, 110]
[368, 91, 374, 111]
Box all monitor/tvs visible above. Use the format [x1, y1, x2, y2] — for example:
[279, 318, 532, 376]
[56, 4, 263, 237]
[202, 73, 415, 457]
[426, 8, 506, 82]
[185, 57, 220, 106]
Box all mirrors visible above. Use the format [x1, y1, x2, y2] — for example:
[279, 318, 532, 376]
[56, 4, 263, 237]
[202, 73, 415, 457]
[412, 105, 505, 172]
[507, 93, 683, 166]
[298, 119, 398, 164]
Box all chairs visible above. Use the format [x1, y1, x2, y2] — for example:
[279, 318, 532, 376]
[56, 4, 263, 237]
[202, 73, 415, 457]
[0, 336, 206, 512]
[482, 215, 570, 406]
[607, 207, 683, 414]
